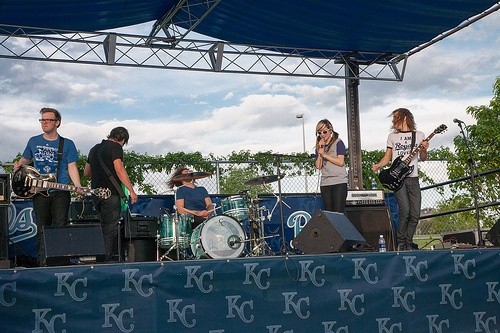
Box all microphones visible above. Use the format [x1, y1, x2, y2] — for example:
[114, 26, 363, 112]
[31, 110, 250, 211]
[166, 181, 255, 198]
[321, 137, 326, 148]
[453, 118, 464, 123]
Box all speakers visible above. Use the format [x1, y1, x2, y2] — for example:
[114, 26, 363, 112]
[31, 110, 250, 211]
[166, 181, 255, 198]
[0, 206, 10, 260]
[486, 218, 500, 247]
[128, 241, 157, 262]
[39, 223, 107, 267]
[291, 209, 366, 255]
[343, 207, 396, 251]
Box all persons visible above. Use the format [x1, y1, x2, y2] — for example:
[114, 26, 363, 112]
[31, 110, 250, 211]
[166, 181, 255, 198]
[13, 107, 85, 264]
[314, 119, 348, 213]
[371, 108, 429, 249]
[84, 127, 138, 262]
[166, 168, 214, 229]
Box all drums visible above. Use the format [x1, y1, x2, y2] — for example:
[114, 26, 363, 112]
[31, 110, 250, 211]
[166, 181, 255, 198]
[190, 214, 245, 260]
[159, 213, 192, 248]
[221, 194, 250, 221]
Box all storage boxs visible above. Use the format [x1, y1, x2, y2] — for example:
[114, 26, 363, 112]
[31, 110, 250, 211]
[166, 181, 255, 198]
[441, 227, 495, 247]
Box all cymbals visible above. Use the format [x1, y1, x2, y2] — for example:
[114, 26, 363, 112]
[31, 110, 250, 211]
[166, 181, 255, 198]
[172, 172, 212, 181]
[247, 175, 284, 185]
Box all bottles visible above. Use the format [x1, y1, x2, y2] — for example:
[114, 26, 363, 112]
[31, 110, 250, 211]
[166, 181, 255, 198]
[371, 178, 377, 190]
[378, 235, 386, 253]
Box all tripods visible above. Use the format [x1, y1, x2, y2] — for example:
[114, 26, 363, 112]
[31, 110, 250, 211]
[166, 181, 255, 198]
[159, 185, 194, 262]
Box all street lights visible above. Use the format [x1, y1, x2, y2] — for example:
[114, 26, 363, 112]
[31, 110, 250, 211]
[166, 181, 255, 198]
[296, 113, 308, 193]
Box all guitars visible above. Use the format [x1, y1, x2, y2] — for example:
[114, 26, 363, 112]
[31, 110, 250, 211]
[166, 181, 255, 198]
[12, 165, 112, 200]
[378, 123, 448, 192]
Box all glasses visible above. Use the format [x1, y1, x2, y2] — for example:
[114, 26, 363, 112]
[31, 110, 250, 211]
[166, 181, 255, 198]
[39, 119, 56, 123]
[318, 129, 330, 134]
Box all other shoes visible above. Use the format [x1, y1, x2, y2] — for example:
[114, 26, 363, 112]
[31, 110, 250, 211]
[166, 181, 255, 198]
[396, 242, 419, 250]
[96, 257, 124, 263]
[46, 260, 69, 266]
